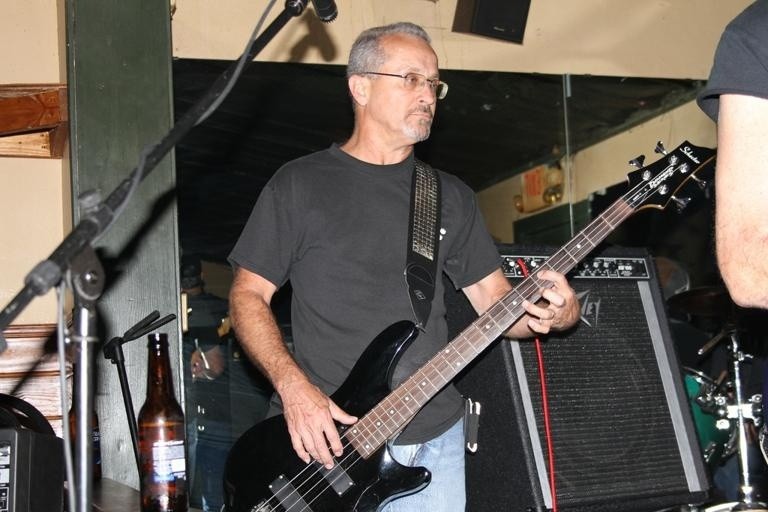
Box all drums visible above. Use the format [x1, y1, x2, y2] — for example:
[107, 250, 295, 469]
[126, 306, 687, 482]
[679, 368, 741, 463]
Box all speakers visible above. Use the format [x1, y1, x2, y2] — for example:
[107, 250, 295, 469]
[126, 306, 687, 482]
[442, 247, 714, 511]
[451, 0, 531, 44]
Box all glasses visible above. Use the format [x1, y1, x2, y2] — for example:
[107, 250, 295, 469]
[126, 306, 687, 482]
[346, 72, 449, 100]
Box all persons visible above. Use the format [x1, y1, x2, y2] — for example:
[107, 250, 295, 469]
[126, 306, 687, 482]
[696, 0, 767, 310]
[226, 22, 580, 512]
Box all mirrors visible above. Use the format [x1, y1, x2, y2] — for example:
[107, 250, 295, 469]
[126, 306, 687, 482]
[166, 46, 768, 512]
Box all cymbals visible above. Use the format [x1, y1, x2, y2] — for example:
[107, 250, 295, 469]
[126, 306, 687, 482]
[651, 256, 690, 304]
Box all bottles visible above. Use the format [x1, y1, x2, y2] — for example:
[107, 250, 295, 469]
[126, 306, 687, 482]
[134, 329, 190, 511]
[68, 362, 104, 487]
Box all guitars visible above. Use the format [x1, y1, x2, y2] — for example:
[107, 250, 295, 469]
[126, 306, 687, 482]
[222, 140, 718, 512]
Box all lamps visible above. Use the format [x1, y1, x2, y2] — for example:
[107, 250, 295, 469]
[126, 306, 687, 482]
[509, 161, 565, 215]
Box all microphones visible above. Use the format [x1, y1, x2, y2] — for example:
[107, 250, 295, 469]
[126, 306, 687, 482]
[310, 0, 338, 23]
